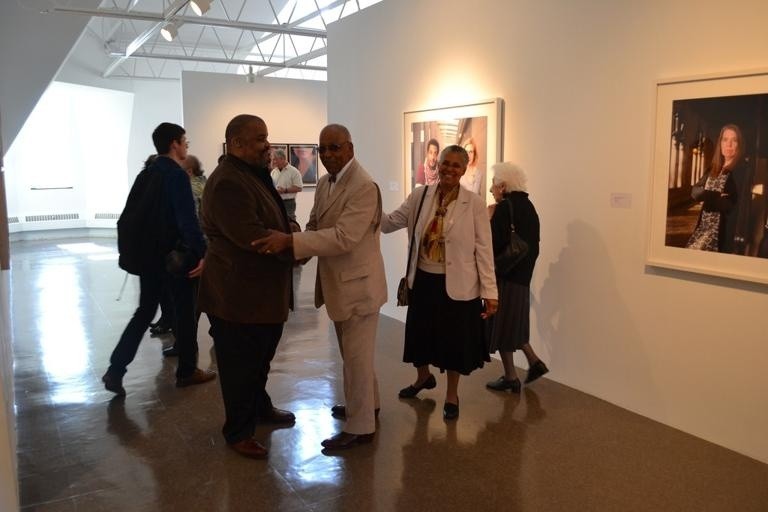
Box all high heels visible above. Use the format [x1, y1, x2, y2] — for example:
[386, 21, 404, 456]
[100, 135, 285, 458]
[487, 376, 521, 392]
[399, 373, 437, 398]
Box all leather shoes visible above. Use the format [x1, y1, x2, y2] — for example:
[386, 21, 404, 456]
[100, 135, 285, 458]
[320, 430, 374, 448]
[443, 395, 460, 419]
[149, 317, 173, 334]
[231, 430, 269, 459]
[163, 345, 178, 357]
[265, 408, 295, 426]
[523, 360, 549, 384]
[102, 373, 128, 396]
[176, 367, 216, 385]
[331, 403, 381, 418]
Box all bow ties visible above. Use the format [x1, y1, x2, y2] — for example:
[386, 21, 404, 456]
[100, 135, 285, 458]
[328, 175, 338, 183]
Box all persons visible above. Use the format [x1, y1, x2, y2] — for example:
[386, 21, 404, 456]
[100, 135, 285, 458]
[485, 162, 549, 393]
[103, 122, 218, 397]
[458, 139, 484, 194]
[251, 124, 387, 449]
[684, 124, 751, 254]
[202, 114, 307, 460]
[269, 149, 303, 219]
[292, 145, 317, 184]
[378, 146, 499, 418]
[414, 139, 441, 189]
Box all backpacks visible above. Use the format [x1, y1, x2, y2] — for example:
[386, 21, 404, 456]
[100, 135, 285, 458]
[117, 154, 166, 275]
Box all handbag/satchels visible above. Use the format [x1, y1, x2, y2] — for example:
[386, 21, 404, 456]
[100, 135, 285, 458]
[490, 231, 528, 273]
[394, 278, 410, 305]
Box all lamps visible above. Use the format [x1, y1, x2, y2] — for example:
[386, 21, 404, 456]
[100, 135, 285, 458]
[158, 0, 213, 43]
[244, 62, 256, 85]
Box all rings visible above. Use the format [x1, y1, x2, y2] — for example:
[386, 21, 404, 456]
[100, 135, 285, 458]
[267, 250, 272, 254]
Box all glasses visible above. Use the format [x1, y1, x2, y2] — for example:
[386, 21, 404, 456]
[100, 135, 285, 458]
[316, 140, 350, 152]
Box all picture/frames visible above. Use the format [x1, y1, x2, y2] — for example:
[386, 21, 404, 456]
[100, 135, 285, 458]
[643, 64, 767, 286]
[288, 142, 321, 187]
[400, 95, 505, 214]
[269, 142, 289, 172]
